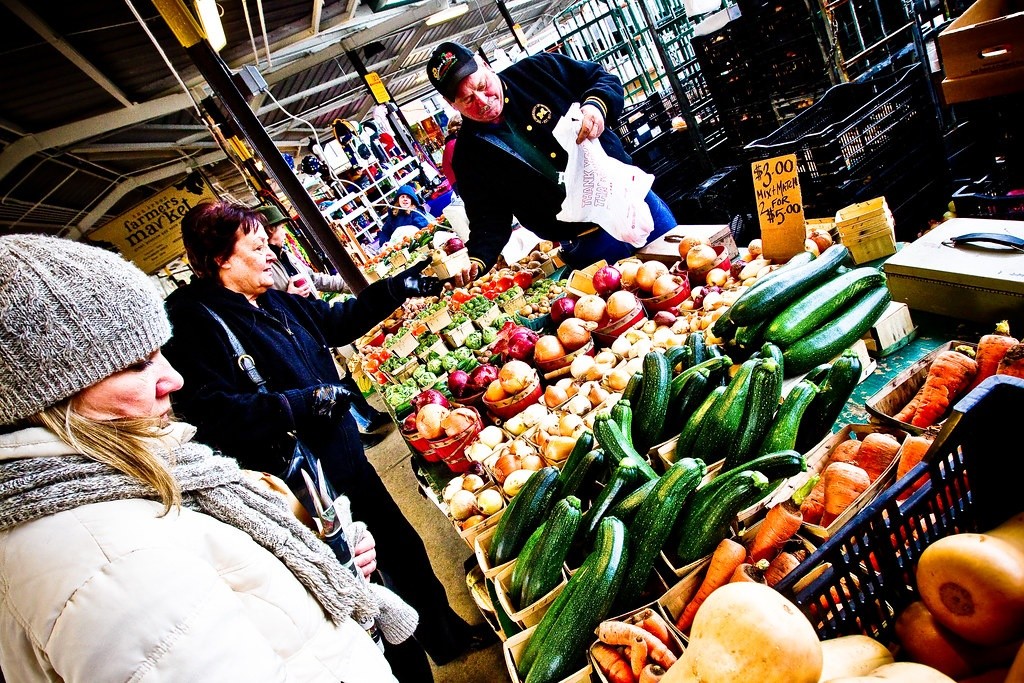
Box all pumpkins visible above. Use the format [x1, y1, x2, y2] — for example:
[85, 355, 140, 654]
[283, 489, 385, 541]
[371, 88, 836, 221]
[656, 511, 1024, 683]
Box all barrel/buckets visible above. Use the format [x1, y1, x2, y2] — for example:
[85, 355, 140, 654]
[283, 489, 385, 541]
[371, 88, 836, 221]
[400, 244, 732, 473]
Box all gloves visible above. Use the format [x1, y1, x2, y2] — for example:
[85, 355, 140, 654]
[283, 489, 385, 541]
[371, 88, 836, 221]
[388, 256, 445, 300]
[285, 384, 359, 422]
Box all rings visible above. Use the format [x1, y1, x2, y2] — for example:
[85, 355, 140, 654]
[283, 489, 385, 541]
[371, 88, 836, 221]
[594, 123, 598, 127]
[471, 273, 477, 277]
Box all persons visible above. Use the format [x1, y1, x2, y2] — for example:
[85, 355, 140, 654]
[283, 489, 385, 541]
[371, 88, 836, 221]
[158, 185, 499, 683]
[426, 42, 677, 291]
[0, 235, 400, 682]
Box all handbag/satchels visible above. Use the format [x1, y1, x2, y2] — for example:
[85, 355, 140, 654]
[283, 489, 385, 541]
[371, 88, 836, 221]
[551, 101, 656, 248]
[251, 441, 333, 514]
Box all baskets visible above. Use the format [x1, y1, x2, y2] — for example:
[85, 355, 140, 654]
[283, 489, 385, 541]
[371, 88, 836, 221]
[778, 376, 1024, 649]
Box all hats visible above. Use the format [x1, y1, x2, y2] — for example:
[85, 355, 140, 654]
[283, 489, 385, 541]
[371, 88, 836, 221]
[251, 205, 291, 227]
[426, 41, 478, 104]
[0, 234, 172, 430]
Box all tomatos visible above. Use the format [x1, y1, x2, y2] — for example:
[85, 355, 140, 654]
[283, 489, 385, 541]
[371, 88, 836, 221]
[364, 270, 531, 384]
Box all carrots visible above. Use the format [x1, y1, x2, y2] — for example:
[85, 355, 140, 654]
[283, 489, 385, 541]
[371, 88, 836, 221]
[735, 320, 1024, 636]
[593, 540, 772, 683]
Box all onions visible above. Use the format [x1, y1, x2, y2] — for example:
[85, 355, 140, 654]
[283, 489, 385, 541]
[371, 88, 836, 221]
[921, 200, 956, 236]
[404, 231, 832, 528]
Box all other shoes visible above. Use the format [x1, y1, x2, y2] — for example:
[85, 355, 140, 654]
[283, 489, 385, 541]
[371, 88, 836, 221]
[464, 622, 498, 654]
[365, 411, 392, 433]
[360, 433, 384, 449]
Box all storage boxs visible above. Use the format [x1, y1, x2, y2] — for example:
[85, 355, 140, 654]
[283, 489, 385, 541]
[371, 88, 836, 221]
[327, 0, 1024, 683]
